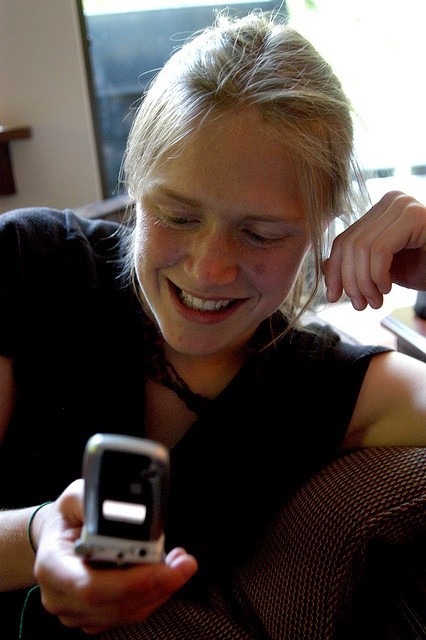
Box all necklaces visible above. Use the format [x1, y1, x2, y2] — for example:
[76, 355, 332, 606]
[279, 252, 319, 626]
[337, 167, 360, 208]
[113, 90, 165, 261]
[142, 337, 212, 420]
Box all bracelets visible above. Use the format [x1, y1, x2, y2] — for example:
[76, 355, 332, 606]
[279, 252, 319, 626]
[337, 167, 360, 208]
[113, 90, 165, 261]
[20, 496, 54, 629]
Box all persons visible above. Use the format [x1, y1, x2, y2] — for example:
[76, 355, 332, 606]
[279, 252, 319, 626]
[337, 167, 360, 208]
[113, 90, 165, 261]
[1, 10, 426, 637]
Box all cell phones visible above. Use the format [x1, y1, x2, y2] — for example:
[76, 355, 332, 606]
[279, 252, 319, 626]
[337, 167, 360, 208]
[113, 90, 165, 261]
[73, 434, 171, 573]
